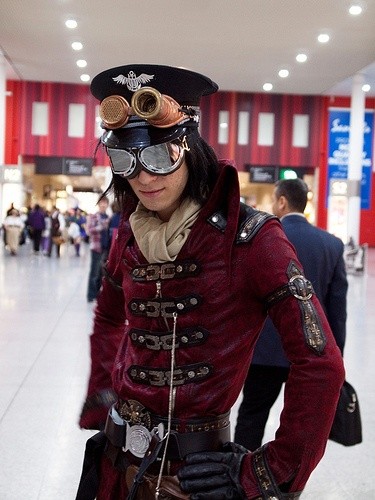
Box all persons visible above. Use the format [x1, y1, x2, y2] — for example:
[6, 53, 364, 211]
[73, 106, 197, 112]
[0, 202, 90, 258]
[87, 196, 119, 303]
[234, 178, 349, 452]
[74, 63, 345, 500]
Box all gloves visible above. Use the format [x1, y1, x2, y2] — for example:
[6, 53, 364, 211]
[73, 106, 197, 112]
[177, 441, 253, 500]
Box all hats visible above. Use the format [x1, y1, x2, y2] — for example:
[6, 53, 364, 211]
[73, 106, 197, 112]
[90, 64, 219, 150]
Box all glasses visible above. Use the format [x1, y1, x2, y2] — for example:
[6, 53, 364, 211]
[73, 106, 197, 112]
[106, 136, 190, 179]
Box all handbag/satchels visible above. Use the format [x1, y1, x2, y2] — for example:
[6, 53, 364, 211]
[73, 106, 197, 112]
[328, 380, 363, 447]
